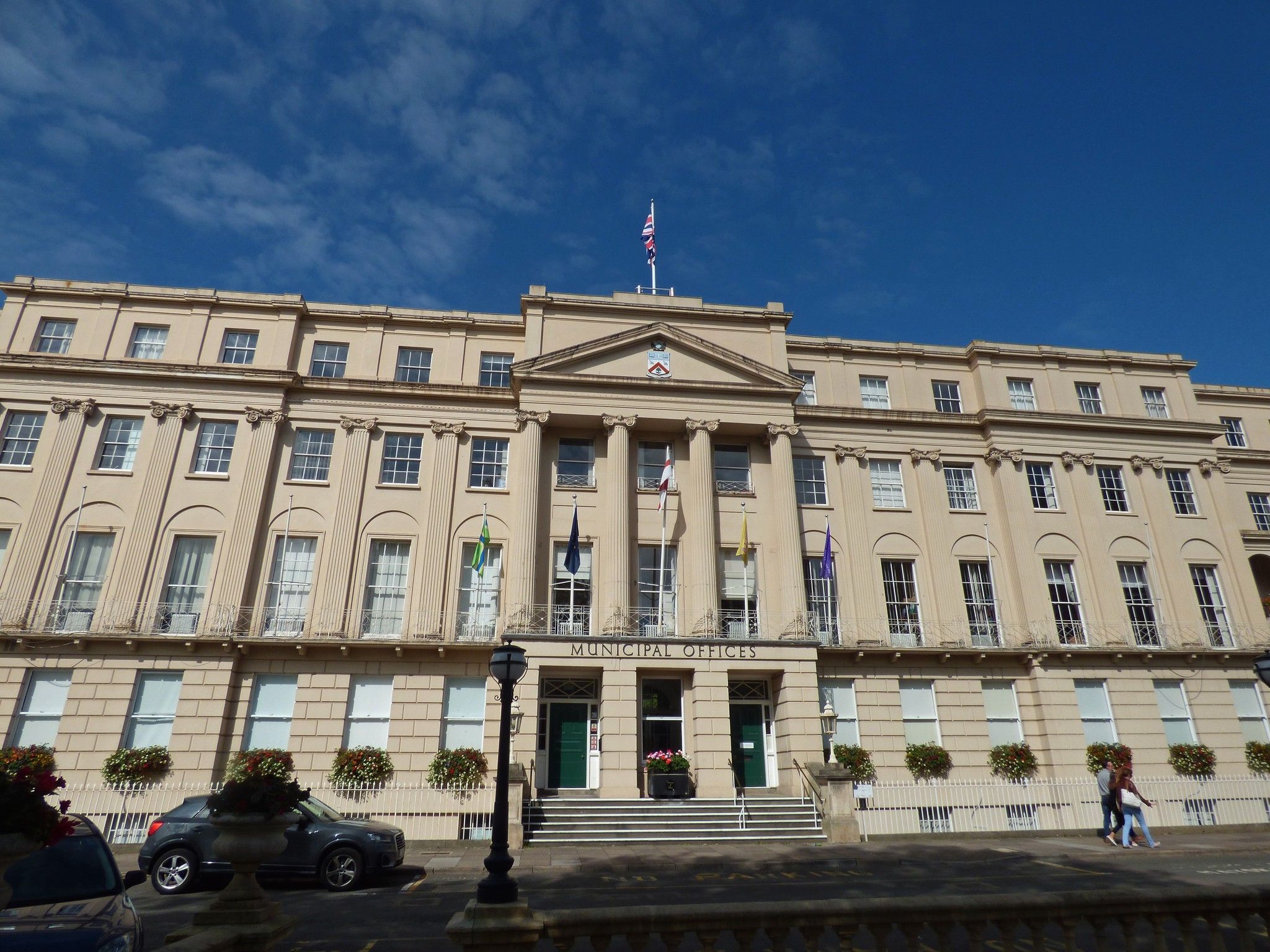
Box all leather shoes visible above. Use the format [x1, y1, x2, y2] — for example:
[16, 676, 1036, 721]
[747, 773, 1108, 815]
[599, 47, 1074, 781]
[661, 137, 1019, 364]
[1104, 837, 1119, 843]
[1131, 835, 1145, 843]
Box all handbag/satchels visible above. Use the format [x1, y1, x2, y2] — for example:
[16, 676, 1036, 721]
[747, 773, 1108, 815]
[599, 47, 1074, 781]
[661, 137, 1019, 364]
[1120, 780, 1142, 808]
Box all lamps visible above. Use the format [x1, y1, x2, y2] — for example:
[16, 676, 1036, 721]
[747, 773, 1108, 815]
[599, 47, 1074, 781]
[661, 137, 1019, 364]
[509, 696, 525, 763]
[819, 698, 841, 762]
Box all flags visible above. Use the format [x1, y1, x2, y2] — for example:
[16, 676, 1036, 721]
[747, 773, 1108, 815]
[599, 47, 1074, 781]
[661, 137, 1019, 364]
[471, 502, 490, 579]
[641, 199, 657, 267]
[657, 445, 673, 514]
[736, 502, 748, 566]
[819, 522, 833, 580]
[564, 495, 580, 576]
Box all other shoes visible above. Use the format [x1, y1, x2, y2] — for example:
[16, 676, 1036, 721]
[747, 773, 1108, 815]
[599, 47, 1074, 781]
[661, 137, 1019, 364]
[1122, 844, 1134, 849]
[1132, 842, 1143, 846]
[1104, 836, 1118, 846]
[1150, 841, 1160, 848]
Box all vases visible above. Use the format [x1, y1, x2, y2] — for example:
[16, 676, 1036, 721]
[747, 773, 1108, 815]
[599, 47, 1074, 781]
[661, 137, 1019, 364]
[647, 764, 689, 796]
[0, 832, 45, 913]
[192, 814, 296, 926]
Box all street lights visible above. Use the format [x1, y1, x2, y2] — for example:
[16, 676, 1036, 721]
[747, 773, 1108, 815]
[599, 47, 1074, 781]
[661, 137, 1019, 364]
[475, 639, 530, 903]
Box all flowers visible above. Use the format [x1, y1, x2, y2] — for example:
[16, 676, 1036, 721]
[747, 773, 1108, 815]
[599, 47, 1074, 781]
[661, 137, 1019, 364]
[905, 739, 956, 780]
[1244, 740, 1270, 773]
[209, 747, 314, 817]
[326, 744, 398, 789]
[425, 746, 489, 792]
[100, 740, 171, 795]
[1086, 740, 1134, 778]
[645, 748, 692, 773]
[1166, 742, 1217, 775]
[826, 745, 877, 783]
[0, 739, 81, 852]
[985, 740, 1040, 787]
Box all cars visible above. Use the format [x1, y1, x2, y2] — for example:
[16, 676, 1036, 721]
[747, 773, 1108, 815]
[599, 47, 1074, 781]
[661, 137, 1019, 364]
[0, 813, 147, 952]
[138, 794, 406, 895]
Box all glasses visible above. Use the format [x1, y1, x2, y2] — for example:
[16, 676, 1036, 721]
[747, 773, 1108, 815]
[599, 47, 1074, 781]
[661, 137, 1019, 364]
[1109, 765, 1114, 768]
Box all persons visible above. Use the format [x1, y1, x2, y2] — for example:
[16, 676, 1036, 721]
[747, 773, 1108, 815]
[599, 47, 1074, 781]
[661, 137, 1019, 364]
[1097, 761, 1160, 849]
